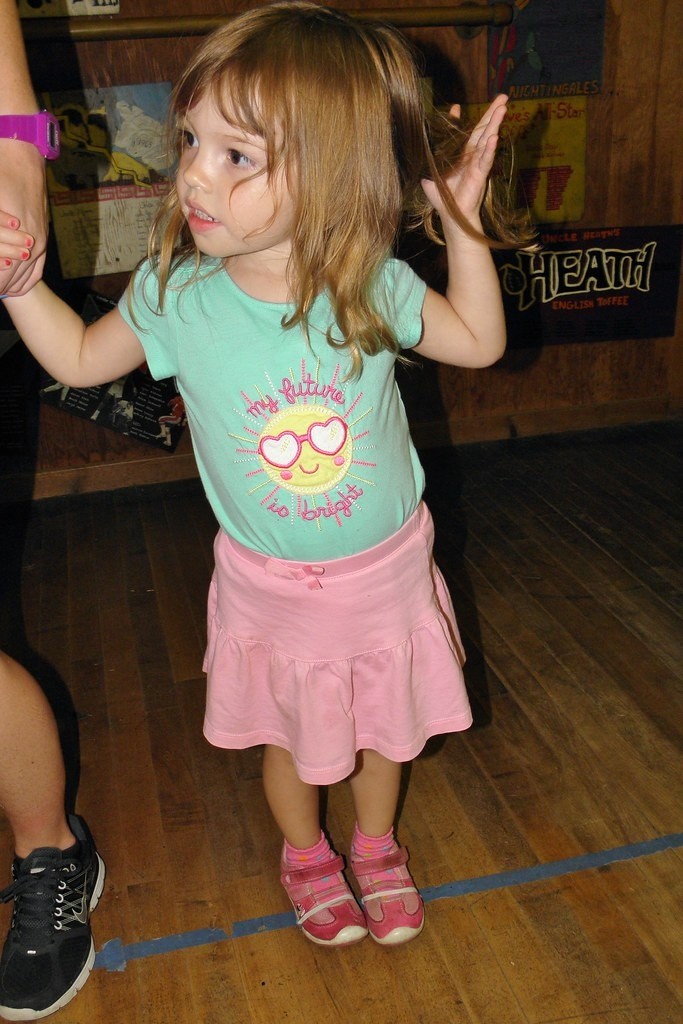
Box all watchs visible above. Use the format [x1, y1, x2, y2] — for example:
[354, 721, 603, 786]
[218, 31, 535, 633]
[0, 108, 60, 160]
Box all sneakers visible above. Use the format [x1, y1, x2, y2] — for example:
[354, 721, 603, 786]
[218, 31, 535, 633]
[0, 813, 106, 1022]
[348, 833, 425, 946]
[279, 846, 369, 948]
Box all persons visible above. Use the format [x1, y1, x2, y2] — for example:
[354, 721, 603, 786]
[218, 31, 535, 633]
[0, 0, 104, 1024]
[0, 0, 543, 946]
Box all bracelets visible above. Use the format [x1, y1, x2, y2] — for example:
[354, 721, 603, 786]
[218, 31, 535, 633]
[0, 295, 9, 300]
